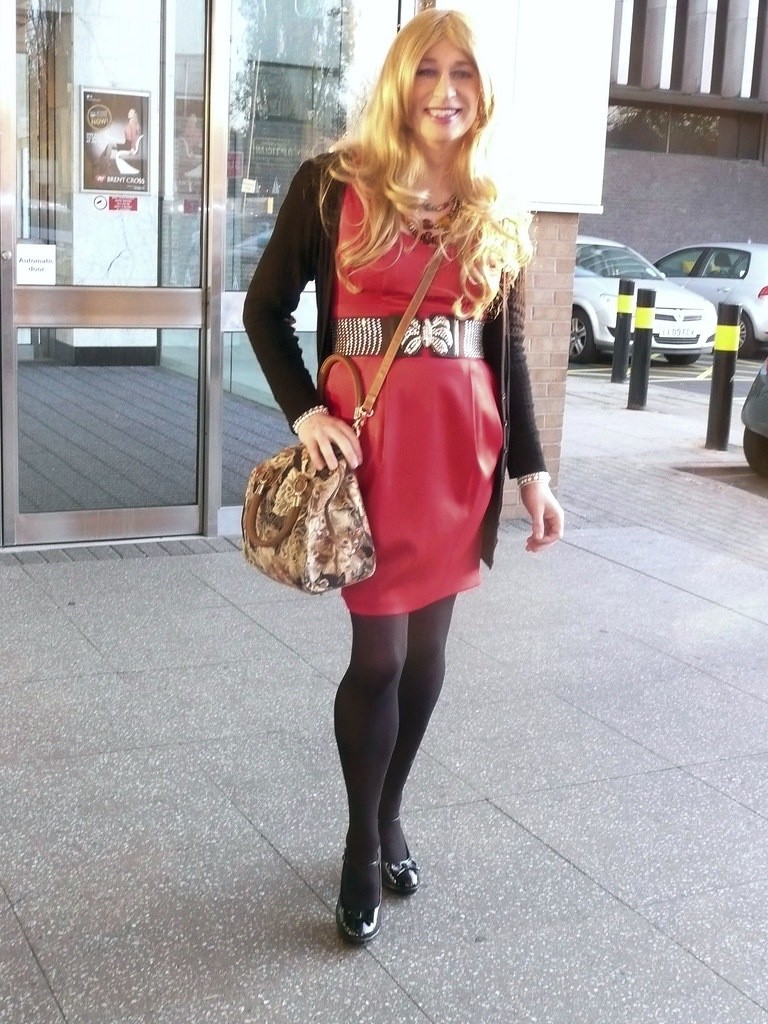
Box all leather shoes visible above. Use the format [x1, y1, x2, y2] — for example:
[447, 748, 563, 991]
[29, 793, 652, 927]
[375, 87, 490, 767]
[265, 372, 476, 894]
[337, 845, 383, 946]
[379, 815, 419, 896]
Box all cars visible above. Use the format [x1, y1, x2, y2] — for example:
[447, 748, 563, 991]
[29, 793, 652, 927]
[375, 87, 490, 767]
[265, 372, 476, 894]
[739, 351, 768, 478]
[652, 238, 768, 360]
[27, 188, 278, 299]
[566, 235, 718, 364]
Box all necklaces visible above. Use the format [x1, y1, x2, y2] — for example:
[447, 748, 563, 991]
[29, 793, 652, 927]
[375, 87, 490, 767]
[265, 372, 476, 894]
[400, 192, 461, 245]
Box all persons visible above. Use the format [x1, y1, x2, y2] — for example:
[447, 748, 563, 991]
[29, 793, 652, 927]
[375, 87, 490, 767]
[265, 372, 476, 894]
[242, 8, 565, 949]
[182, 114, 202, 150]
[93, 108, 140, 169]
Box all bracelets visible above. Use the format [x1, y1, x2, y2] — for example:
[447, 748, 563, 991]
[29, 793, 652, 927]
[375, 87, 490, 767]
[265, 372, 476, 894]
[291, 405, 330, 434]
[517, 472, 551, 489]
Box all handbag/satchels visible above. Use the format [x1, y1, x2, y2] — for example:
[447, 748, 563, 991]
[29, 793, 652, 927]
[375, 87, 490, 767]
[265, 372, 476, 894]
[240, 349, 378, 596]
